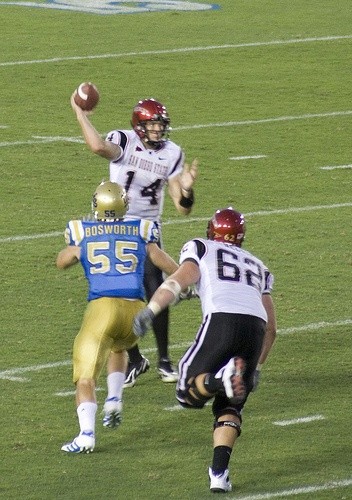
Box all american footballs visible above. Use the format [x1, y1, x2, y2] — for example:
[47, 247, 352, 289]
[74, 82, 99, 110]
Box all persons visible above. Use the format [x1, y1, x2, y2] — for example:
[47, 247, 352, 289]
[131, 208, 277, 492]
[55, 182, 195, 455]
[69, 86, 200, 392]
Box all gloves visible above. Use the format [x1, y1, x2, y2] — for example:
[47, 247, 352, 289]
[178, 287, 192, 301]
[132, 308, 154, 337]
[250, 370, 259, 392]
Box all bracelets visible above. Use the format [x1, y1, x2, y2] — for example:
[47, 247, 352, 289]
[178, 188, 195, 209]
[255, 362, 263, 372]
[147, 300, 163, 316]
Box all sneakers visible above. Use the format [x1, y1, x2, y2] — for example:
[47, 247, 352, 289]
[214, 356, 246, 404]
[61, 430, 96, 453]
[122, 354, 150, 389]
[157, 359, 178, 382]
[208, 467, 233, 494]
[102, 396, 123, 429]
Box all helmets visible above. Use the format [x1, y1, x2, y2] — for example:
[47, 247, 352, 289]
[131, 99, 169, 152]
[92, 182, 129, 219]
[207, 209, 245, 247]
